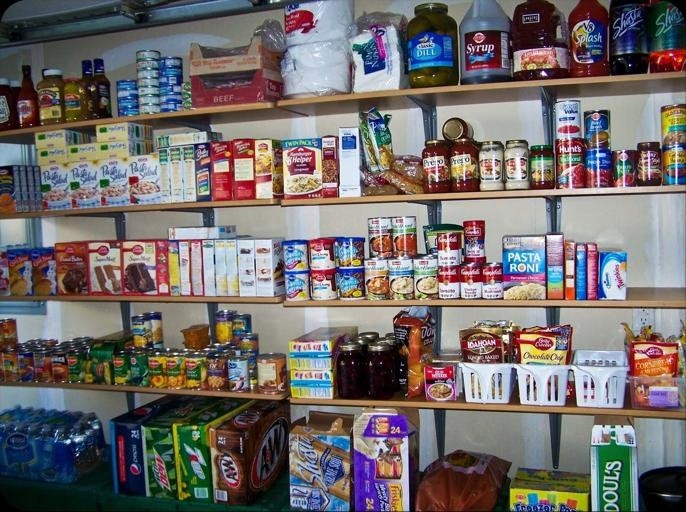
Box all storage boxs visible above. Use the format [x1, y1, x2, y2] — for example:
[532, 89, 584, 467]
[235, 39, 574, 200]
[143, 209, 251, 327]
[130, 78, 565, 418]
[591, 411, 638, 512]
[189, 35, 283, 75]
[509, 467, 590, 511]
[189, 69, 283, 109]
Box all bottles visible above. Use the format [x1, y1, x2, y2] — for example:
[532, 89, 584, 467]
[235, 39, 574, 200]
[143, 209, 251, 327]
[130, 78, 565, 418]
[40, 67, 51, 79]
[91, 57, 112, 118]
[9, 81, 22, 130]
[0, 78, 15, 130]
[17, 64, 39, 129]
[78, 59, 96, 120]
[0, 405, 104, 484]
[566, 0, 611, 77]
[646, 1, 685, 71]
[610, 0, 650, 75]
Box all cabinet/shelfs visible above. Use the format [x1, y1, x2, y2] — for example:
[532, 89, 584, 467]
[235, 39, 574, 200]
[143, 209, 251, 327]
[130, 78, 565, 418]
[0, 72, 686, 512]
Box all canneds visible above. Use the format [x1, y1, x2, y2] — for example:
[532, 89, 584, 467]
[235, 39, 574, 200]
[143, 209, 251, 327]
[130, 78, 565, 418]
[413, 256, 437, 300]
[458, 262, 481, 298]
[479, 141, 504, 191]
[231, 314, 253, 332]
[242, 335, 258, 351]
[659, 104, 686, 145]
[115, 353, 131, 385]
[131, 316, 155, 347]
[134, 50, 159, 116]
[585, 150, 612, 186]
[150, 344, 194, 354]
[558, 140, 585, 187]
[336, 341, 362, 399]
[421, 141, 452, 192]
[662, 141, 685, 185]
[634, 142, 664, 186]
[116, 80, 139, 117]
[281, 240, 311, 271]
[310, 270, 336, 301]
[361, 256, 389, 299]
[113, 351, 132, 385]
[377, 338, 398, 376]
[308, 238, 336, 270]
[582, 109, 613, 148]
[368, 218, 392, 258]
[615, 150, 636, 185]
[36, 67, 64, 125]
[186, 356, 203, 390]
[147, 312, 163, 344]
[435, 232, 459, 268]
[390, 217, 419, 258]
[0, 318, 93, 386]
[368, 344, 390, 395]
[206, 354, 227, 391]
[483, 263, 503, 299]
[260, 355, 288, 395]
[407, 1, 458, 86]
[333, 237, 365, 266]
[62, 76, 87, 122]
[243, 353, 256, 386]
[283, 272, 307, 300]
[448, 138, 480, 190]
[157, 56, 182, 112]
[227, 357, 249, 392]
[531, 144, 558, 188]
[213, 309, 233, 344]
[360, 331, 379, 340]
[131, 353, 147, 386]
[462, 221, 486, 261]
[147, 357, 165, 386]
[439, 266, 458, 298]
[552, 98, 585, 137]
[501, 141, 529, 190]
[389, 258, 414, 299]
[441, 118, 474, 140]
[164, 355, 184, 388]
[337, 266, 364, 299]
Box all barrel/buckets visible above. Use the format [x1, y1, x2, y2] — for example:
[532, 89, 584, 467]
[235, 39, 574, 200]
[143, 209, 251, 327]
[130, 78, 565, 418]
[508, 0, 571, 80]
[458, 1, 512, 83]
